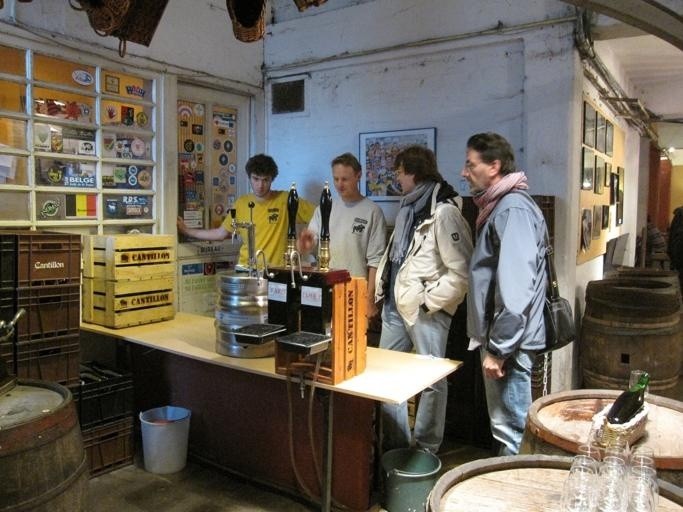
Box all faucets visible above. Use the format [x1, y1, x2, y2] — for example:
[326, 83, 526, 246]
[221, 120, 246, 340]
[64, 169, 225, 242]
[289, 251, 311, 288]
[254, 249, 275, 286]
[226, 208, 240, 245]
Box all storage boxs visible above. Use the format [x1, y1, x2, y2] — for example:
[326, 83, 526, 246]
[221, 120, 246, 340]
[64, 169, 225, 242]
[81, 234, 175, 280]
[82, 276, 174, 329]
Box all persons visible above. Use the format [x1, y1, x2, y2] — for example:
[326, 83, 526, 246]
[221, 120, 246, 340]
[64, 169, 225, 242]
[645, 212, 666, 258]
[666, 205, 681, 296]
[372, 146, 473, 458]
[295, 151, 387, 343]
[176, 154, 315, 271]
[458, 130, 547, 458]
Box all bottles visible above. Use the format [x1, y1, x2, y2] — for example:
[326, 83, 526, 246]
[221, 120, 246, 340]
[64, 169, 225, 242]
[608, 373, 650, 424]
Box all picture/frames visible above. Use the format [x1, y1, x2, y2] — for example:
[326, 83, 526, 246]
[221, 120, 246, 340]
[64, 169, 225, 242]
[359, 128, 436, 202]
[582, 100, 624, 248]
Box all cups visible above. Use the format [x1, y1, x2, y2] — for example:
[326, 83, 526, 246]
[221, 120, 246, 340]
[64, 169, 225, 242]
[564, 437, 659, 512]
[628, 370, 649, 403]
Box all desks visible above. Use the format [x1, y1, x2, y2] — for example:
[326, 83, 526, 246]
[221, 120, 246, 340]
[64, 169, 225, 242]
[80, 313, 465, 512]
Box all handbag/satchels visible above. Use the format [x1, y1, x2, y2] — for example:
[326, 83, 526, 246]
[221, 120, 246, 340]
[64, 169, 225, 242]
[536, 294, 578, 357]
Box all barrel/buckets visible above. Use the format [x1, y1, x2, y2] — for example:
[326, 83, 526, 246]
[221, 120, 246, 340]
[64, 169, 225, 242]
[0, 376, 89, 512]
[214, 269, 277, 358]
[617, 266, 680, 281]
[425, 455, 683, 512]
[578, 277, 683, 402]
[517, 388, 683, 488]
[380, 447, 442, 512]
[138, 406, 192, 475]
